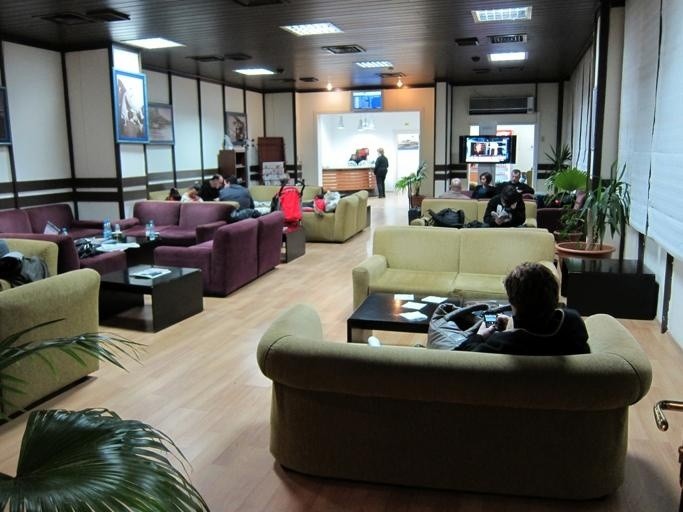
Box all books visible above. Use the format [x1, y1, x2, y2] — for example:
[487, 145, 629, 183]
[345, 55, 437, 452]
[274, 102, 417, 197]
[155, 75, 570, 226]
[491, 210, 509, 222]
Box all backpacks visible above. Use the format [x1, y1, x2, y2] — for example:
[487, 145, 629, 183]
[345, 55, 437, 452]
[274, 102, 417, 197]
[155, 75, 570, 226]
[424, 208, 464, 227]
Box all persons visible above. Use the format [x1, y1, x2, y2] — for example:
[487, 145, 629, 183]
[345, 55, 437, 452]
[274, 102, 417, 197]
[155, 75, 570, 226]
[251, 192, 324, 209]
[438, 177, 471, 200]
[497, 169, 534, 199]
[181, 185, 204, 202]
[164, 188, 182, 201]
[198, 172, 223, 200]
[483, 184, 526, 227]
[472, 171, 497, 199]
[218, 175, 255, 210]
[452, 261, 590, 355]
[372, 148, 388, 198]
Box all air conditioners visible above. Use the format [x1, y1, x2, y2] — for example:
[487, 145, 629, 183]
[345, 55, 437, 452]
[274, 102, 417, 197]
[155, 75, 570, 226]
[467, 96, 534, 116]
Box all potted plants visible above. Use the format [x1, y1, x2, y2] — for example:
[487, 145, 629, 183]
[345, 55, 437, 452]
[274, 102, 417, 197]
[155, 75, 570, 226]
[394, 161, 428, 208]
[544, 162, 632, 266]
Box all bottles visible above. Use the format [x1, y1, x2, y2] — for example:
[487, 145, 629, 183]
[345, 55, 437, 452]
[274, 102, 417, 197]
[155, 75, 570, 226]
[114, 223, 119, 233]
[148, 219, 155, 240]
[144, 224, 149, 236]
[103, 216, 112, 240]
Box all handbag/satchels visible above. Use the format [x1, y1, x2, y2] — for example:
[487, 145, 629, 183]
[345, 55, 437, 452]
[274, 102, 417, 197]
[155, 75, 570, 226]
[76, 238, 101, 258]
[427, 303, 484, 349]
[20, 256, 48, 281]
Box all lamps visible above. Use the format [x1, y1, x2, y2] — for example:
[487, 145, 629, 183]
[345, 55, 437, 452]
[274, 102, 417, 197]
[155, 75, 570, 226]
[336, 116, 374, 133]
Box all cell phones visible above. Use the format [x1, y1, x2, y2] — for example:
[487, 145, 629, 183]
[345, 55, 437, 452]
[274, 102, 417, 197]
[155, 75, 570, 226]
[483, 311, 498, 329]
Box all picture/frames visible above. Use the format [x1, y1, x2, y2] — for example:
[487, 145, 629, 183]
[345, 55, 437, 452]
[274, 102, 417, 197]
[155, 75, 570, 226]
[0, 86, 12, 147]
[225, 111, 248, 146]
[112, 67, 151, 145]
[148, 102, 175, 146]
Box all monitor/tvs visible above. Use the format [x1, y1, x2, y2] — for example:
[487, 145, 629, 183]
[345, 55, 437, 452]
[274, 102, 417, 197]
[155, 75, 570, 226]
[458, 136, 517, 164]
[351, 90, 384, 111]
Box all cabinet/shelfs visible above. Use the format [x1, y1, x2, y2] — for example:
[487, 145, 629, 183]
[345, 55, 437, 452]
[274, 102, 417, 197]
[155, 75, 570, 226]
[217, 148, 249, 190]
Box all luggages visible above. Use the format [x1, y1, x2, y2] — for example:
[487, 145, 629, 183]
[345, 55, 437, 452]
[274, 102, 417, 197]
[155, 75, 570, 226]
[408, 206, 420, 225]
